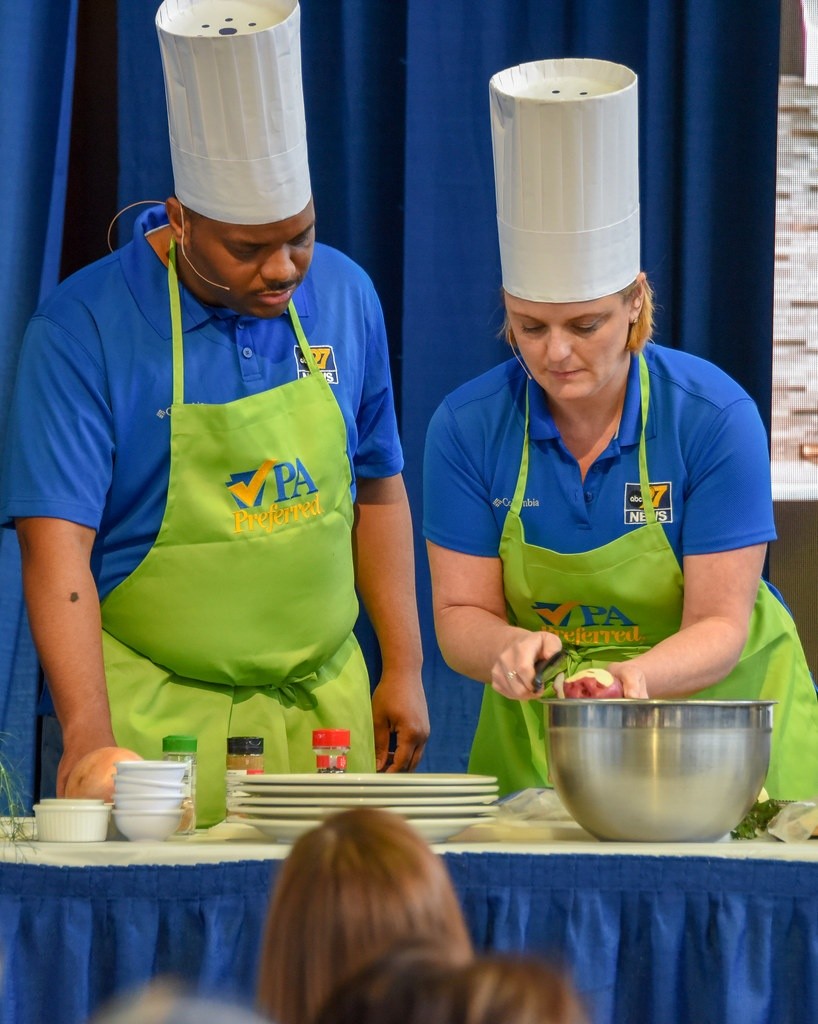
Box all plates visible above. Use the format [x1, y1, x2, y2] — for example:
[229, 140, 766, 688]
[223, 770, 506, 844]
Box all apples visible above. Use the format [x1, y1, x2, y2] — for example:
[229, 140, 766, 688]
[562, 667, 624, 699]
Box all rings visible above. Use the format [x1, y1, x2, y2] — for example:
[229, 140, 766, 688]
[506, 670, 517, 681]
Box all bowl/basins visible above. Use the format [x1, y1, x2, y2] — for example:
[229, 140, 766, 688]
[112, 758, 188, 842]
[32, 797, 119, 843]
[536, 695, 780, 841]
[0, 815, 38, 841]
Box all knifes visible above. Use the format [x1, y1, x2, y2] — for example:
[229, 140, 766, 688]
[530, 644, 574, 691]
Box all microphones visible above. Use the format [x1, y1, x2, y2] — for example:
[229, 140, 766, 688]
[508, 332, 532, 380]
[178, 203, 231, 291]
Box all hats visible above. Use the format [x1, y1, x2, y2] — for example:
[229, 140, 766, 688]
[156, 0, 312, 227]
[489, 57, 643, 307]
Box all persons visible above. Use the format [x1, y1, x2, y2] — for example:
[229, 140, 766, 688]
[421, 266, 817, 800]
[90, 807, 598, 1024]
[0, 192, 431, 831]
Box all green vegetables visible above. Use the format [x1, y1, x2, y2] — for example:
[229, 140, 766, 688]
[730, 799, 784, 840]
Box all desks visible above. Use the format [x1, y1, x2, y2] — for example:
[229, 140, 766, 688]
[1, 836, 817, 1024]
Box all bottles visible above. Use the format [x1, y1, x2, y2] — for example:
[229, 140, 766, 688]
[226, 733, 268, 822]
[162, 733, 197, 835]
[312, 727, 351, 774]
